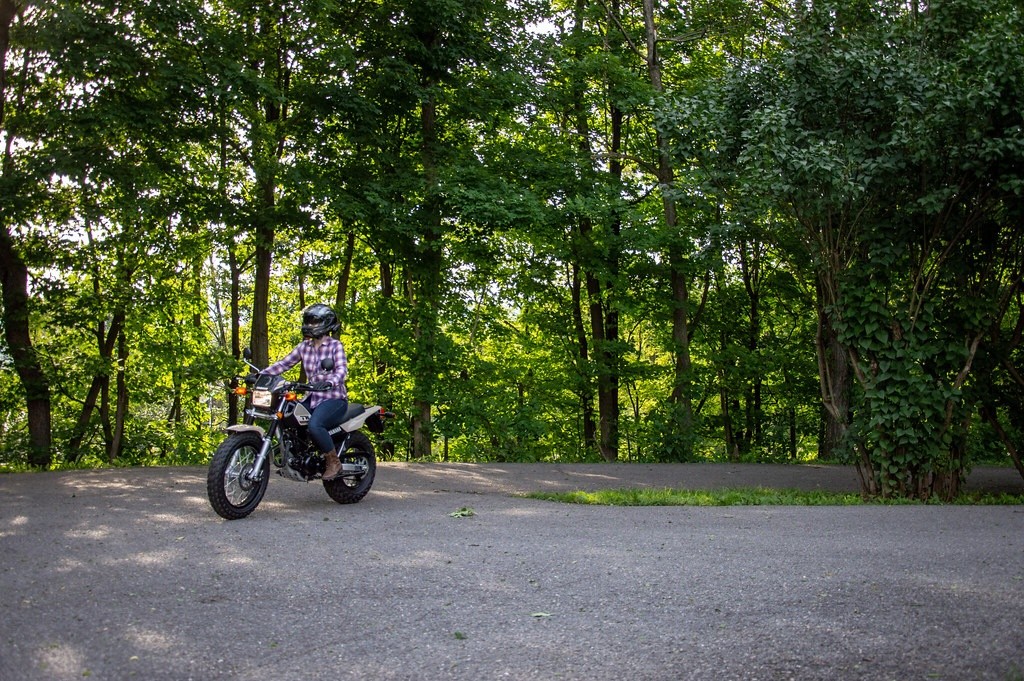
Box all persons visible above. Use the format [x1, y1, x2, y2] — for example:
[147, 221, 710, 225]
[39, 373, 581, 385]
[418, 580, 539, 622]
[245, 304, 349, 481]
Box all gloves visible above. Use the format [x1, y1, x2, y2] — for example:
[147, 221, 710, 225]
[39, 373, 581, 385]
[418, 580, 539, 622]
[313, 382, 332, 391]
[245, 374, 256, 384]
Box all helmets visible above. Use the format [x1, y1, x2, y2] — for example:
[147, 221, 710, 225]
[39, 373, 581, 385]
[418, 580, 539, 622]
[302, 304, 337, 338]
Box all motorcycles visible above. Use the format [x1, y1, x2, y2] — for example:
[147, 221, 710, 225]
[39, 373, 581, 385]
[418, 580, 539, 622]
[207, 347, 397, 521]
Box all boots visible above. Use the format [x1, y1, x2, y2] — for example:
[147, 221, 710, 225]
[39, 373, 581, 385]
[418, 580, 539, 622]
[322, 447, 343, 481]
